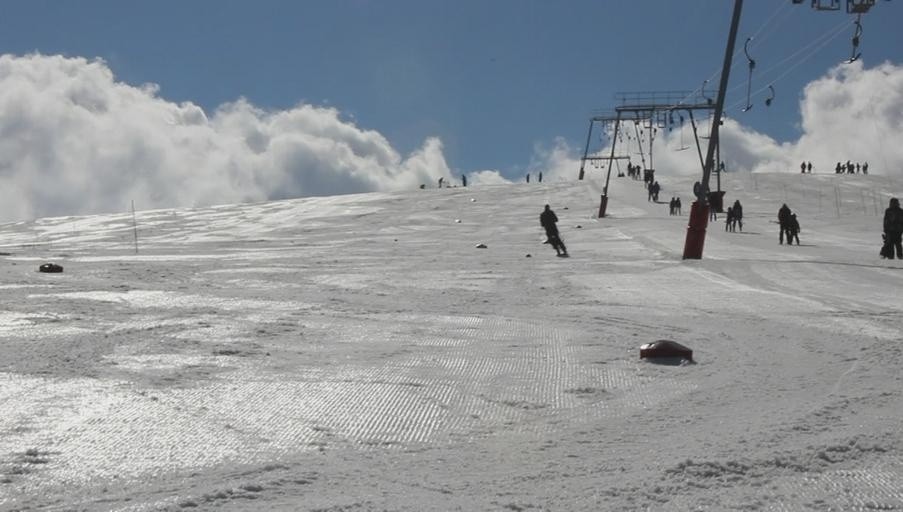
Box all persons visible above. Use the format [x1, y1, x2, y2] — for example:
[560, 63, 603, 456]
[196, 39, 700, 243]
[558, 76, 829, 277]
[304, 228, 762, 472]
[835, 160, 869, 175]
[526, 173, 529, 183]
[625, 160, 744, 233]
[807, 162, 811, 172]
[790, 214, 800, 246]
[539, 204, 567, 256]
[538, 172, 542, 182]
[777, 204, 791, 245]
[801, 162, 806, 172]
[438, 177, 443, 188]
[460, 174, 466, 187]
[883, 198, 903, 261]
